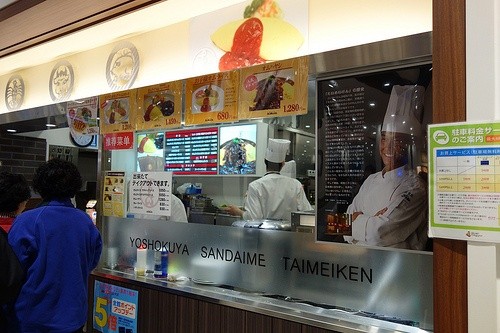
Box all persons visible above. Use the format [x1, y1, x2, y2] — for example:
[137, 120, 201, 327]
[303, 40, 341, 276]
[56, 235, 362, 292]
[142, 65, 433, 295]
[7, 157, 102, 333]
[343, 111, 432, 253]
[127, 170, 188, 223]
[222, 147, 315, 222]
[1, 172, 32, 234]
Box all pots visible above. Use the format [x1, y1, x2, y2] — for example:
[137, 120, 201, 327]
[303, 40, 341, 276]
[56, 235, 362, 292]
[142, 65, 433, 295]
[232, 219, 292, 292]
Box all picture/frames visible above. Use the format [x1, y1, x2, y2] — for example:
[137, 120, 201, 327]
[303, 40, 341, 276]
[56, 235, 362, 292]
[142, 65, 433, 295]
[315, 60, 433, 255]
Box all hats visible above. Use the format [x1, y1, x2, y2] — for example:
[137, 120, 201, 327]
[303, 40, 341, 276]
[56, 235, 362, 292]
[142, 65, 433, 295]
[265, 138, 291, 164]
[382, 85, 425, 137]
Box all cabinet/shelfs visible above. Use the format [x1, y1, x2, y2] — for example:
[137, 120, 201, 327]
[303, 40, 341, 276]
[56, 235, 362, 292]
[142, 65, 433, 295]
[88, 272, 409, 333]
[280, 126, 315, 205]
[188, 209, 245, 227]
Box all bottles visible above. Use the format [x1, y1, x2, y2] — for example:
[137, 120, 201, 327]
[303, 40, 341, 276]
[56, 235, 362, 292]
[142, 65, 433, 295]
[154, 247, 169, 278]
[135, 242, 147, 276]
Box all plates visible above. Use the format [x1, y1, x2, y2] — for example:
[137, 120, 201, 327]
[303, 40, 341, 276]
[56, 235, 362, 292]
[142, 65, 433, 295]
[192, 85, 224, 114]
